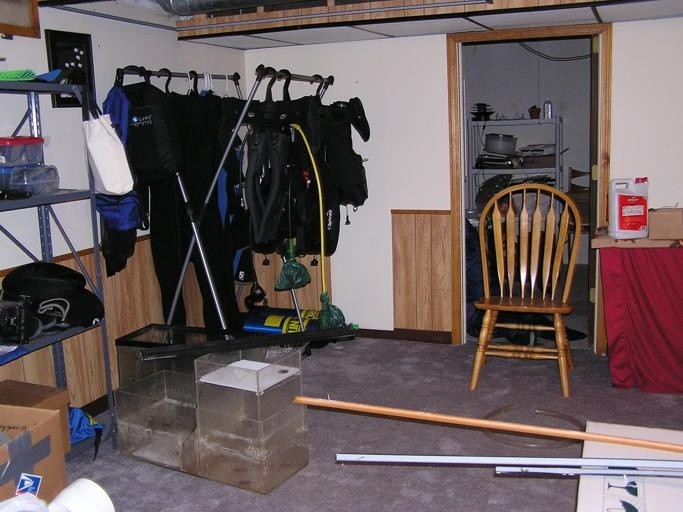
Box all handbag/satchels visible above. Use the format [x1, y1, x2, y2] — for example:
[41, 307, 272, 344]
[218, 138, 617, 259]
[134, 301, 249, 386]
[1, 262, 105, 329]
[81, 88, 136, 196]
[1, 294, 31, 346]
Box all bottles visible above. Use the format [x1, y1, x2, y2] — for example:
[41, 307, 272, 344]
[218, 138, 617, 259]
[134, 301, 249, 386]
[543, 99, 552, 119]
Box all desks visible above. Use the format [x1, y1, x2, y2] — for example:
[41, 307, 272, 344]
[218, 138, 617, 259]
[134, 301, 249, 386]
[593, 235, 683, 386]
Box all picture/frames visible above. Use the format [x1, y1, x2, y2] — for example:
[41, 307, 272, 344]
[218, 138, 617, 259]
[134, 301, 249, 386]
[45, 29, 97, 109]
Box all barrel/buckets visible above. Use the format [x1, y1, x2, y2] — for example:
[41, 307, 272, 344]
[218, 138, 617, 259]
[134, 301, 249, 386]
[608, 175, 651, 240]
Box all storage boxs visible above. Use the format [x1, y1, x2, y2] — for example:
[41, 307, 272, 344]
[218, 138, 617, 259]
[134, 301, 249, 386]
[573, 419, 682, 512]
[1, 404, 71, 507]
[1, 378, 72, 456]
[0, 136, 60, 195]
[647, 207, 683, 241]
[109, 322, 311, 497]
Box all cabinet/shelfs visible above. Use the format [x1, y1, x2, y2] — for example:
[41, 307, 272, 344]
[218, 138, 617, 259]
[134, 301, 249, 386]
[466, 114, 564, 272]
[0, 77, 120, 460]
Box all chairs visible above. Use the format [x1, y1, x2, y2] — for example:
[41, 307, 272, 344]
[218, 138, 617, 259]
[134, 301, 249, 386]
[567, 167, 589, 271]
[467, 182, 584, 398]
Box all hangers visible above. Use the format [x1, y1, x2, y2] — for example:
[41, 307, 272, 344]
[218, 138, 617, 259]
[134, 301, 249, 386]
[111, 61, 353, 126]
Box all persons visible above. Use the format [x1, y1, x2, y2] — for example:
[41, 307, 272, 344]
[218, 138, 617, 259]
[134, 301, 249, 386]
[464, 218, 588, 351]
[487, 197, 555, 297]
